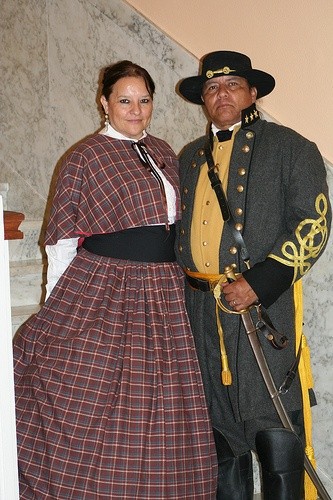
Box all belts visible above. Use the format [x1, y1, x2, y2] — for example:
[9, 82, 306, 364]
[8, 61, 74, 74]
[186, 273, 218, 291]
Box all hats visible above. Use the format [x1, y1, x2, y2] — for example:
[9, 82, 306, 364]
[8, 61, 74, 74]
[179, 51, 275, 105]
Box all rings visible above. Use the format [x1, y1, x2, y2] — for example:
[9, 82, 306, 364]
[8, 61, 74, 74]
[233, 300, 238, 306]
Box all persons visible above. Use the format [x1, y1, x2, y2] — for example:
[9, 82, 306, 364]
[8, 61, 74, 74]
[13, 60, 243, 500]
[173, 50, 333, 500]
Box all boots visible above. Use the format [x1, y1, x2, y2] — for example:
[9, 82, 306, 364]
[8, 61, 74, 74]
[256, 428, 306, 500]
[212, 429, 253, 500]
